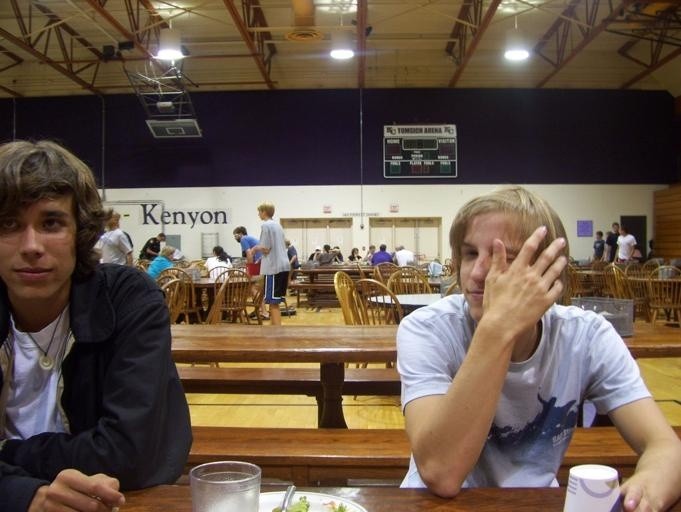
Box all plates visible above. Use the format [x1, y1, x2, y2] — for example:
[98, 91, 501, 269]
[260, 489, 369, 512]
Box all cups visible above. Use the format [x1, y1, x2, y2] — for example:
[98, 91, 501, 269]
[563, 462, 623, 509]
[191, 460, 262, 512]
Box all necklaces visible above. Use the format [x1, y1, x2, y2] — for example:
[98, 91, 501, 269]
[16, 307, 65, 370]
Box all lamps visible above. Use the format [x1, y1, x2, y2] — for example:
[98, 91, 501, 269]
[505, 4, 529, 61]
[156, 9, 182, 59]
[330, 7, 353, 60]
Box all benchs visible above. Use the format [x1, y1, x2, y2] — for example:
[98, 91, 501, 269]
[182, 426, 681, 486]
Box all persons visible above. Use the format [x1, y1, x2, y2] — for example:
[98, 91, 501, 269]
[147, 245, 179, 279]
[398, 184, 681, 511]
[593, 221, 656, 269]
[98, 210, 135, 265]
[203, 246, 232, 320]
[304, 240, 418, 283]
[232, 225, 269, 322]
[249, 199, 293, 326]
[286, 238, 299, 288]
[0, 139, 192, 512]
[138, 232, 167, 260]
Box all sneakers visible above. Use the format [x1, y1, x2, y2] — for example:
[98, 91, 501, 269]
[249, 310, 270, 320]
